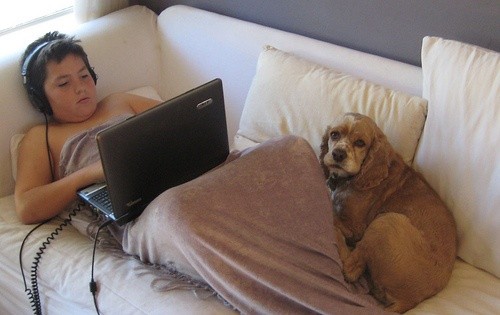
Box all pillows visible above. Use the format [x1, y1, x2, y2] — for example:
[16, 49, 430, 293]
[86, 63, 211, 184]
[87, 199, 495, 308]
[9, 86, 165, 179]
[231, 43, 429, 170]
[410, 34, 500, 280]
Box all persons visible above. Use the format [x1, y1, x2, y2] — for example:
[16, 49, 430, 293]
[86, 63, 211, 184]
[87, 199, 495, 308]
[15, 30, 164, 225]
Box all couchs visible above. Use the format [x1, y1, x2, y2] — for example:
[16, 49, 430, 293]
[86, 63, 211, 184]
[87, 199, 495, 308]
[0, 4, 499, 314]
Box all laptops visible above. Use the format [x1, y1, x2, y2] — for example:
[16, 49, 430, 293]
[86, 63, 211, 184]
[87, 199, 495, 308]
[76, 78, 229, 227]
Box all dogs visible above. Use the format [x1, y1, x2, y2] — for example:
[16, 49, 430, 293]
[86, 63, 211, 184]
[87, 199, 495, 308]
[319, 112, 457, 314]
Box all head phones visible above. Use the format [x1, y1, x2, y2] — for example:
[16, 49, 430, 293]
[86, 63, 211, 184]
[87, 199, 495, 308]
[21, 40, 97, 116]
